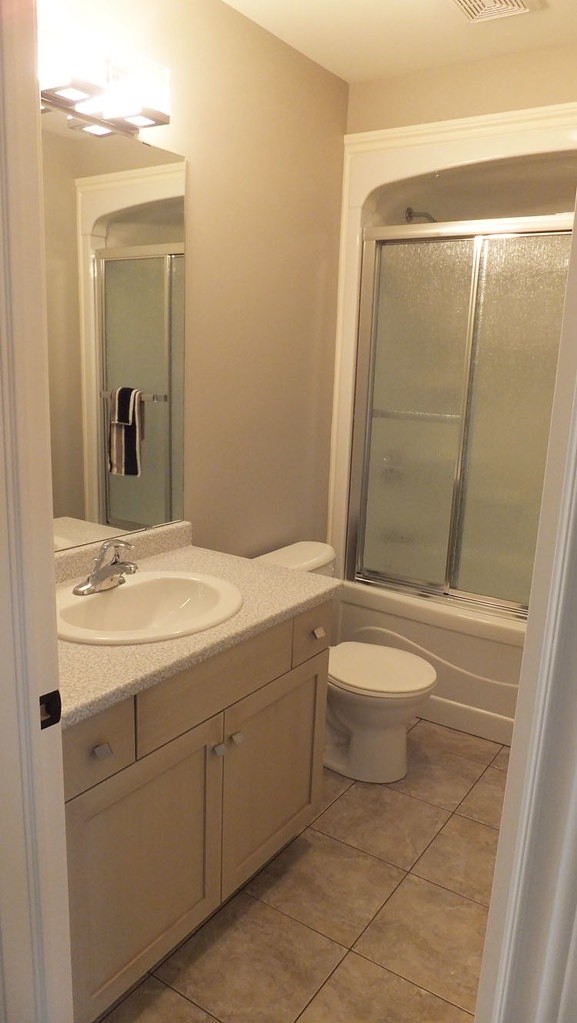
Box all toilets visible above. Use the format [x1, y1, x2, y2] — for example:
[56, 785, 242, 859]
[249, 539, 437, 784]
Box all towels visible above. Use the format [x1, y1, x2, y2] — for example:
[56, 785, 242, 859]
[104, 386, 146, 479]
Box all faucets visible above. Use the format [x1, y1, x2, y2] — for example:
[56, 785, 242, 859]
[71, 539, 140, 596]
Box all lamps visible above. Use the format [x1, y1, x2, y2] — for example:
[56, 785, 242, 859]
[39, 80, 106, 108]
[41, 104, 52, 114]
[68, 117, 116, 138]
[103, 106, 171, 132]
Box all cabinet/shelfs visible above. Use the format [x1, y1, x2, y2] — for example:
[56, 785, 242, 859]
[55, 519, 346, 1023]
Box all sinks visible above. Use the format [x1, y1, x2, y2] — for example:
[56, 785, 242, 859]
[56, 571, 242, 646]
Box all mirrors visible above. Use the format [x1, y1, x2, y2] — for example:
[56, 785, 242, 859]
[38, 99, 188, 553]
